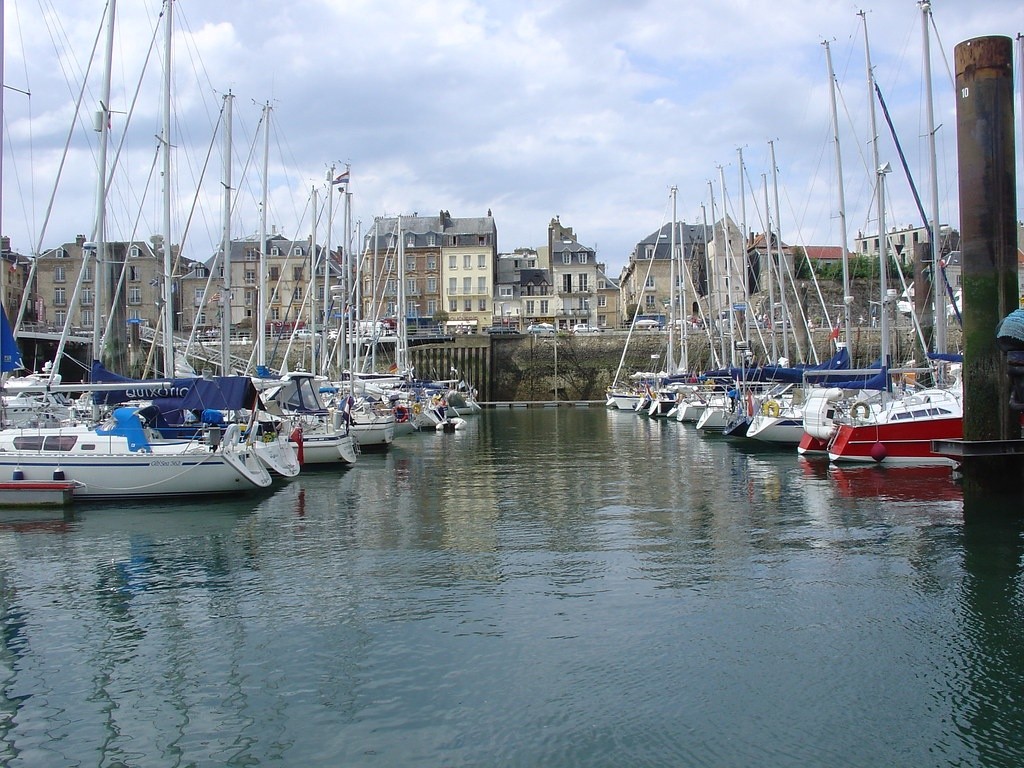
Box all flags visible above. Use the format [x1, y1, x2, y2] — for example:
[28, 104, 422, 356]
[209, 291, 220, 303]
[291, 421, 305, 465]
[829, 324, 840, 340]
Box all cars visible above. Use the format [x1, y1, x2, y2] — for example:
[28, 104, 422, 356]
[527, 325, 555, 334]
[487, 326, 521, 334]
[574, 323, 600, 333]
[194, 332, 209, 342]
[294, 329, 320, 340]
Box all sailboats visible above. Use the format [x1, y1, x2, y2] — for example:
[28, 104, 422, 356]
[604, 0, 964, 470]
[1, 3, 482, 510]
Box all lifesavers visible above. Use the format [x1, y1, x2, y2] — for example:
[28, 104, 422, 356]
[764, 400, 779, 417]
[246, 420, 259, 446]
[222, 423, 240, 446]
[851, 401, 870, 419]
[392, 405, 408, 423]
[704, 380, 715, 389]
[412, 403, 421, 415]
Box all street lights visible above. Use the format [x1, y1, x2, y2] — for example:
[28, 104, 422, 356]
[498, 302, 504, 335]
[585, 300, 590, 334]
[414, 304, 420, 337]
